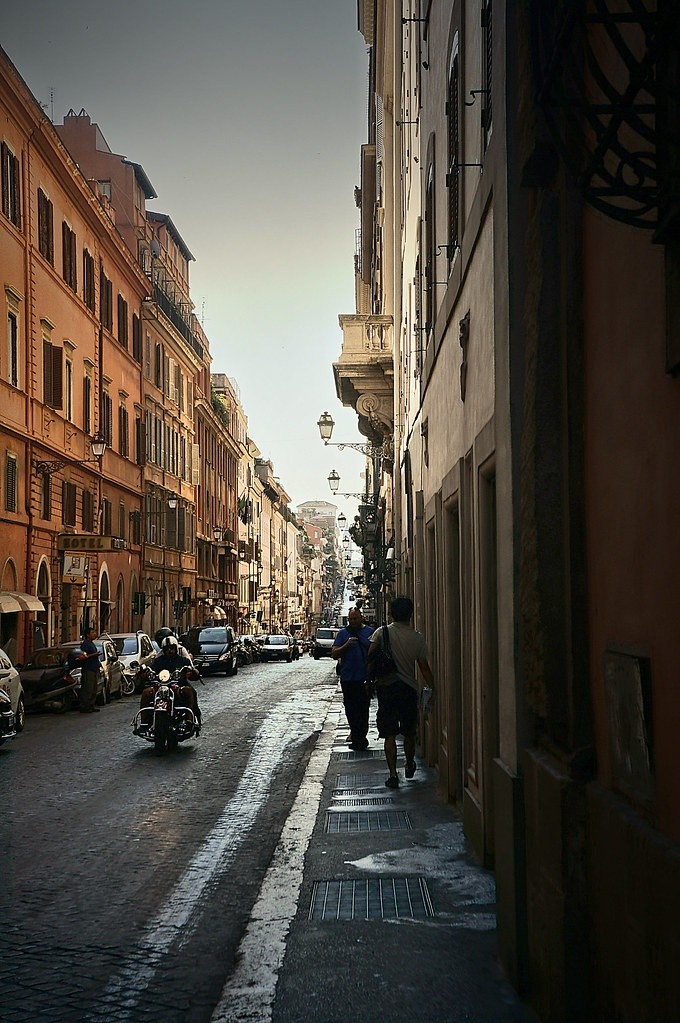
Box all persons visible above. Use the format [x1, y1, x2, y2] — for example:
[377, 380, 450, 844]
[80, 627, 103, 713]
[0, 596, 22, 613]
[332, 609, 375, 751]
[133, 627, 199, 735]
[367, 597, 435, 788]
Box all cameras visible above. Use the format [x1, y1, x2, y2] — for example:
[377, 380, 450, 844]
[348, 637, 358, 644]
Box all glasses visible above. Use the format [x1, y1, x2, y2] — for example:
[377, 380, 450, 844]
[164, 646, 174, 651]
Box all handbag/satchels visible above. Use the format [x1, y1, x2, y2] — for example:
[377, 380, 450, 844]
[374, 625, 393, 665]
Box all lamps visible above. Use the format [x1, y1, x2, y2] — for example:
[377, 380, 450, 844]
[36, 431, 107, 478]
[327, 470, 378, 505]
[345, 555, 365, 569]
[338, 512, 361, 532]
[342, 535, 363, 552]
[196, 525, 221, 548]
[348, 571, 354, 581]
[129, 491, 178, 522]
[232, 548, 245, 561]
[257, 577, 276, 591]
[240, 565, 263, 580]
[317, 411, 393, 461]
[263, 589, 279, 600]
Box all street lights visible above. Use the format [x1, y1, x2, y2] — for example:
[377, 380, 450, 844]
[268, 584, 272, 634]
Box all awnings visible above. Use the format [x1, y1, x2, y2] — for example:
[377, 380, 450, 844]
[215, 606, 227, 619]
[206, 607, 221, 620]
[10, 592, 45, 611]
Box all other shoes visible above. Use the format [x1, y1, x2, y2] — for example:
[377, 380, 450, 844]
[133, 726, 145, 735]
[385, 776, 398, 788]
[92, 707, 100, 712]
[80, 709, 92, 713]
[405, 760, 416, 778]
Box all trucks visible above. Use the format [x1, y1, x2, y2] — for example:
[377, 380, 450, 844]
[311, 628, 341, 661]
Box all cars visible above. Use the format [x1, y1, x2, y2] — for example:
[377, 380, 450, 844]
[0, 648, 26, 745]
[239, 632, 309, 664]
[61, 639, 123, 708]
[24, 645, 107, 707]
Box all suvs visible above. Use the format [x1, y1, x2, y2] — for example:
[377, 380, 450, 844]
[178, 624, 239, 677]
[95, 630, 157, 678]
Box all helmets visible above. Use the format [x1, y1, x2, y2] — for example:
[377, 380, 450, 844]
[162, 636, 178, 653]
[155, 627, 173, 649]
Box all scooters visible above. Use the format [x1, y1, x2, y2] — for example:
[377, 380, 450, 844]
[235, 637, 262, 668]
[307, 641, 314, 657]
[13, 659, 78, 715]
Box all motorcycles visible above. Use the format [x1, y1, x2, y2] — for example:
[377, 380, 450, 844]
[129, 660, 206, 758]
[118, 660, 135, 696]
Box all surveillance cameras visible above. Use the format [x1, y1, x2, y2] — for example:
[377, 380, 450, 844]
[386, 548, 395, 560]
